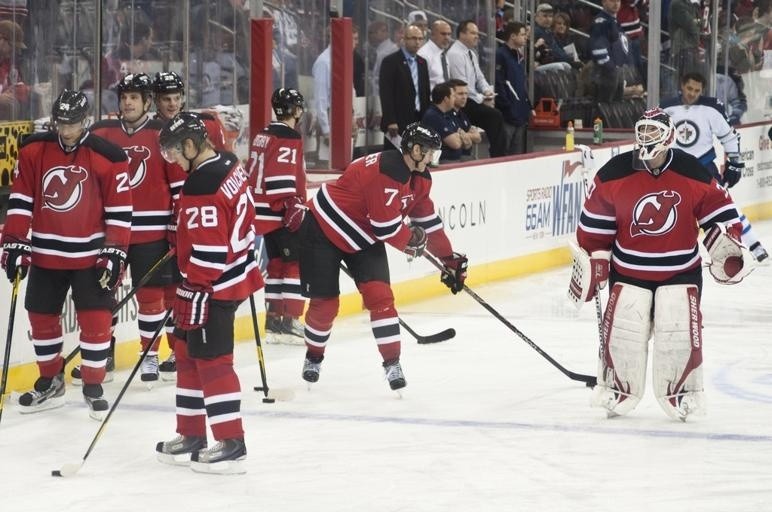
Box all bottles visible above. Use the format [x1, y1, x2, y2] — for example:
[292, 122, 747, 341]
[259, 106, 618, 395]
[593, 118, 602, 146]
[565, 120, 574, 151]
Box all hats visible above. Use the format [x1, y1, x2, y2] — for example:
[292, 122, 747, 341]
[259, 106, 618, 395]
[0, 21, 27, 51]
[407, 10, 427, 26]
[536, 4, 555, 13]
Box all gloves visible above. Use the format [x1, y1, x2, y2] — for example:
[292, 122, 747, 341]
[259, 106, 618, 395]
[172, 286, 211, 329]
[92, 245, 128, 297]
[565, 237, 612, 311]
[406, 224, 426, 259]
[284, 198, 305, 232]
[166, 218, 177, 249]
[723, 161, 744, 187]
[1, 236, 31, 282]
[442, 257, 467, 294]
[708, 234, 757, 286]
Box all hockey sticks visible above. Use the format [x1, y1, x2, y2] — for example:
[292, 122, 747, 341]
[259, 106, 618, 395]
[421, 250, 597, 383]
[9, 247, 175, 403]
[52, 307, 172, 476]
[338, 262, 455, 344]
[250, 293, 294, 400]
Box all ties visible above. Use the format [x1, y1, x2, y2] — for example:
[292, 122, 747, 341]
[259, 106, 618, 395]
[409, 58, 417, 89]
[441, 53, 449, 81]
[469, 50, 477, 80]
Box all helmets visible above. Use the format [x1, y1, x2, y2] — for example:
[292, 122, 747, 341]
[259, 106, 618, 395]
[153, 71, 185, 94]
[159, 111, 206, 149]
[53, 91, 89, 124]
[402, 124, 442, 152]
[117, 73, 151, 93]
[272, 87, 305, 119]
[634, 107, 678, 161]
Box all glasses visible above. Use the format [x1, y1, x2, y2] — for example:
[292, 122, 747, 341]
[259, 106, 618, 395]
[403, 36, 424, 40]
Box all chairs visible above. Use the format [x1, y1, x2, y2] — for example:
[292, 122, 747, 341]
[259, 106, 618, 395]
[472, 0, 688, 132]
[0, 0, 252, 122]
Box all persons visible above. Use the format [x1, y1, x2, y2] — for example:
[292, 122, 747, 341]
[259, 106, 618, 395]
[753, 0, 772, 50]
[589, 0, 632, 103]
[553, 11, 588, 68]
[0, 21, 39, 122]
[297, 121, 468, 391]
[1, 91, 130, 421]
[666, 0, 705, 77]
[663, 77, 768, 262]
[368, 18, 400, 128]
[101, 24, 151, 90]
[496, 20, 536, 156]
[571, 107, 750, 424]
[151, 72, 185, 124]
[449, 79, 482, 163]
[422, 82, 462, 164]
[381, 27, 431, 147]
[534, 5, 584, 72]
[418, 23, 451, 87]
[69, 72, 186, 385]
[247, 90, 309, 345]
[616, 0, 643, 68]
[711, 65, 748, 125]
[447, 20, 507, 158]
[311, 22, 366, 170]
[156, 112, 265, 477]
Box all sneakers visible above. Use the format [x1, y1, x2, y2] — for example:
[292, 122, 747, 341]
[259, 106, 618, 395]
[20, 359, 65, 406]
[156, 433, 207, 455]
[383, 358, 405, 390]
[189, 437, 246, 463]
[282, 316, 306, 334]
[750, 241, 768, 262]
[82, 383, 108, 411]
[139, 351, 159, 381]
[158, 350, 179, 372]
[265, 315, 302, 338]
[302, 351, 325, 381]
[71, 335, 115, 378]
